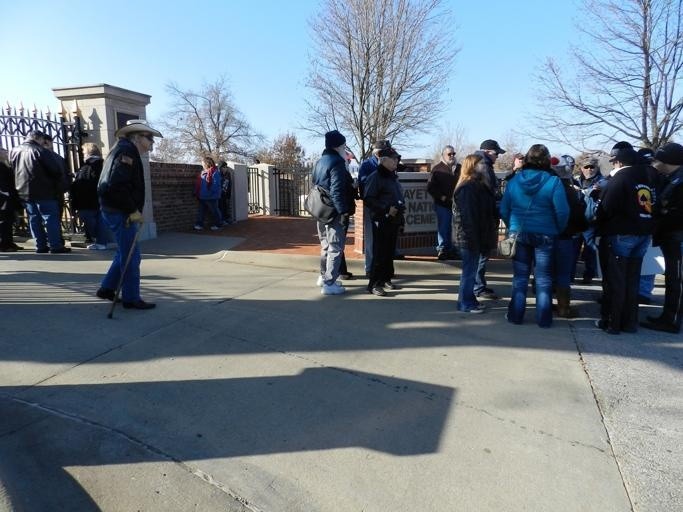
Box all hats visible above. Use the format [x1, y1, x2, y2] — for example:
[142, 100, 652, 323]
[378, 148, 400, 158]
[479, 140, 505, 154]
[608, 142, 683, 165]
[371, 141, 390, 149]
[515, 153, 524, 159]
[562, 155, 575, 166]
[325, 130, 345, 150]
[114, 119, 163, 138]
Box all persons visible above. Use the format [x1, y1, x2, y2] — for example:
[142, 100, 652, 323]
[336, 151, 360, 280]
[8, 130, 70, 253]
[216, 161, 231, 226]
[95, 119, 163, 309]
[41, 134, 75, 245]
[67, 143, 106, 250]
[0, 149, 23, 252]
[311, 130, 349, 294]
[192, 157, 224, 230]
[358, 140, 391, 278]
[428, 146, 461, 259]
[363, 148, 407, 296]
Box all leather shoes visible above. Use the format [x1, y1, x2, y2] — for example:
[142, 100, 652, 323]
[58, 247, 70, 253]
[437, 250, 449, 260]
[316, 271, 404, 296]
[0, 243, 17, 252]
[96, 287, 122, 303]
[470, 274, 683, 334]
[37, 246, 48, 253]
[10, 243, 22, 250]
[123, 299, 156, 309]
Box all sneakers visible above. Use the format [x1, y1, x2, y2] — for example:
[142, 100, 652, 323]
[193, 220, 228, 230]
[87, 244, 105, 250]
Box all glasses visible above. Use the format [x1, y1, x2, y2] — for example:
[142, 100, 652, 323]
[583, 165, 595, 169]
[140, 134, 152, 141]
[448, 152, 456, 156]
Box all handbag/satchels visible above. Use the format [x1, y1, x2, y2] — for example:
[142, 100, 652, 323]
[497, 238, 516, 259]
[304, 185, 338, 225]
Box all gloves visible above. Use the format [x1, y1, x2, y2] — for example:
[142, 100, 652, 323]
[129, 209, 143, 224]
[339, 212, 349, 226]
